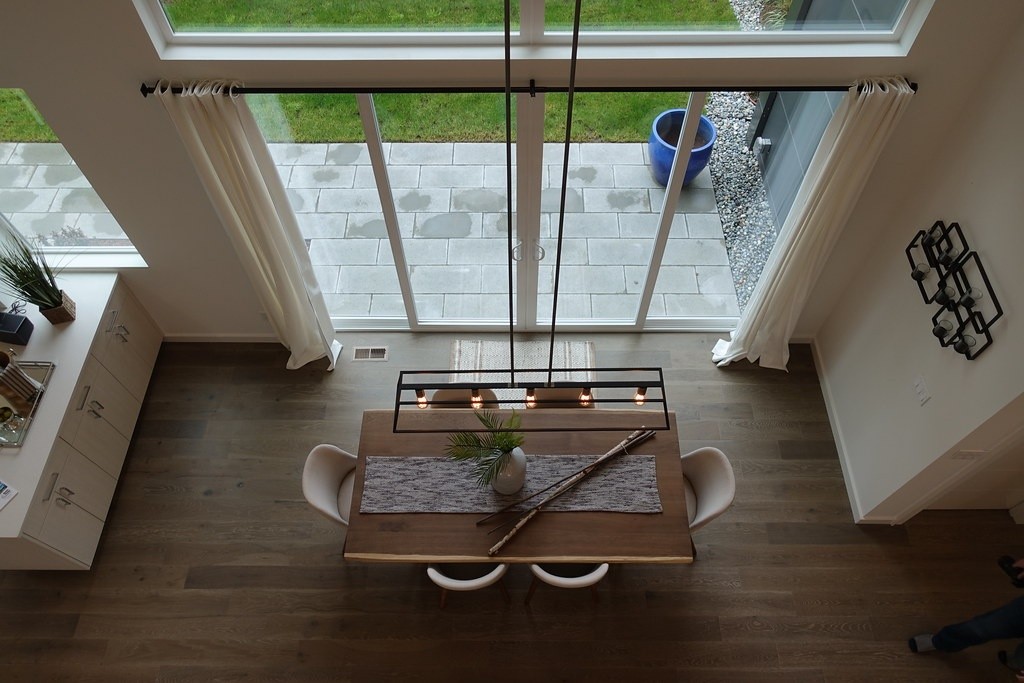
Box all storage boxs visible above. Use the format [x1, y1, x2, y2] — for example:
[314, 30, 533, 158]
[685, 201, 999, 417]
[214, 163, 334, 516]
[0, 312, 34, 346]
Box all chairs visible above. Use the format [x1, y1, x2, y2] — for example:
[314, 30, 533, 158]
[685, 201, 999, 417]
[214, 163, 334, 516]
[302, 444, 357, 558]
[679, 447, 736, 557]
[425, 562, 610, 591]
[431, 386, 595, 409]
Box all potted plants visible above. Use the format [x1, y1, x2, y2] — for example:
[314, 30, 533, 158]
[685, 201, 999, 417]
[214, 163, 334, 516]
[0, 229, 76, 325]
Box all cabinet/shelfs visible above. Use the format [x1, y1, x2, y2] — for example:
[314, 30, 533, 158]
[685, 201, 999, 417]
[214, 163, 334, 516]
[0, 276, 165, 570]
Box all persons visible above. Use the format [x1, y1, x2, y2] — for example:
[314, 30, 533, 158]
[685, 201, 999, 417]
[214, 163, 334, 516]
[909, 556, 1024, 683]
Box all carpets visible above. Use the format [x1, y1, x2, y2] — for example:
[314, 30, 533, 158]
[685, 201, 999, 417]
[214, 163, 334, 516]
[449, 339, 597, 410]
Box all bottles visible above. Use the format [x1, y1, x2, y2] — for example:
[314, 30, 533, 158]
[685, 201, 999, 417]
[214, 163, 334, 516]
[0, 406, 26, 443]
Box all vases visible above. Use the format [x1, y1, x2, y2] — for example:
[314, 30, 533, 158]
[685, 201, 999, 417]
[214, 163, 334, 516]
[649, 108, 718, 186]
[491, 446, 527, 495]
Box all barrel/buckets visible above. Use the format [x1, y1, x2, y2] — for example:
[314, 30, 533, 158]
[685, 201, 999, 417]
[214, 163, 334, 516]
[646, 109, 717, 186]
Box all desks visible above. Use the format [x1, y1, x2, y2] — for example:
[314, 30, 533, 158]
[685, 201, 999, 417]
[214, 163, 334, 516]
[345, 409, 693, 564]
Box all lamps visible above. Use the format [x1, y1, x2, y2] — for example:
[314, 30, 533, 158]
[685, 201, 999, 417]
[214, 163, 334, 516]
[393, 0, 670, 434]
[905, 218, 1003, 361]
